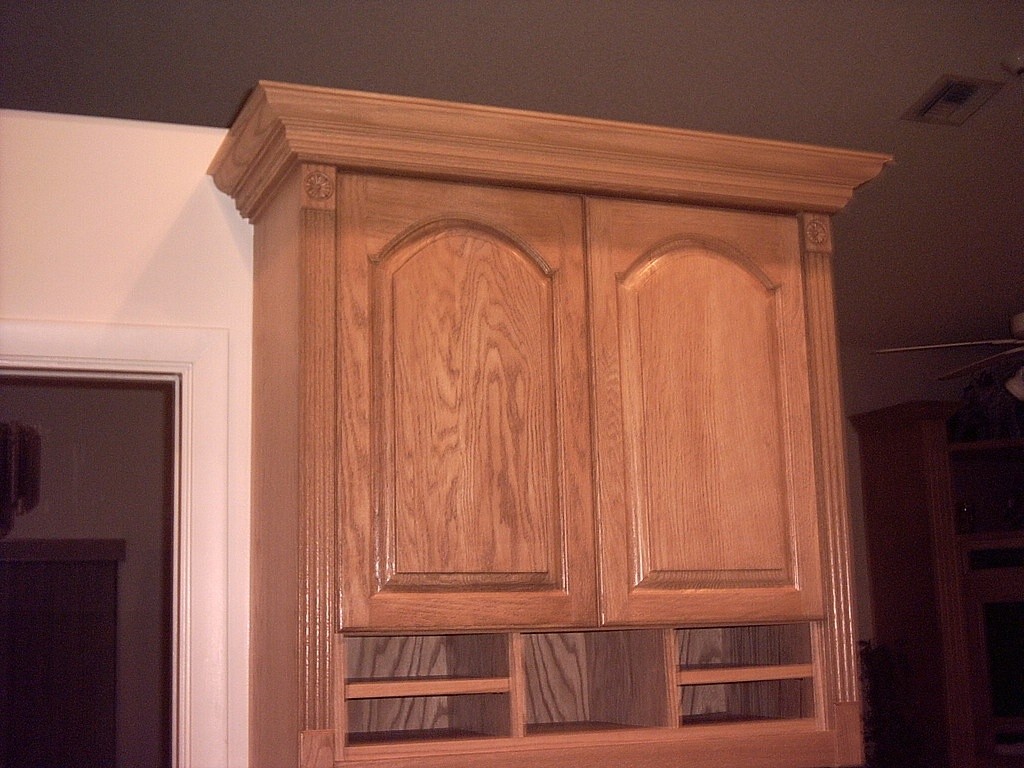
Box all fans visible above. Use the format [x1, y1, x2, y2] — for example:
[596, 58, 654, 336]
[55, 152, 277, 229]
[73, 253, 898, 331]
[876, 313, 1024, 380]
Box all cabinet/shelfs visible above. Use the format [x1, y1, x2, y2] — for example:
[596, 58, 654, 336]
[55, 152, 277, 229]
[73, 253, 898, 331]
[206, 81, 893, 768]
[847, 400, 1024, 768]
